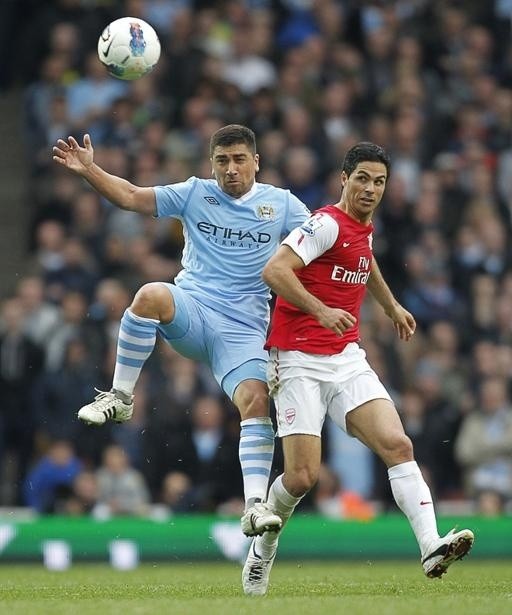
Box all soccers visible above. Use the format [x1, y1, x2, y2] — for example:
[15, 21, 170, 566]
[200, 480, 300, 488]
[97, 17, 161, 80]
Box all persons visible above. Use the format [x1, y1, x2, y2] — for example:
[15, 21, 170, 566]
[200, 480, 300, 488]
[1, 0, 510, 522]
[52, 125, 314, 538]
[241, 141, 474, 597]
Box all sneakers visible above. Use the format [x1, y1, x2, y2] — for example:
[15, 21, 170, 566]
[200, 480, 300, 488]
[242, 538, 277, 596]
[240, 504, 282, 536]
[77, 388, 134, 424]
[420, 528, 474, 579]
